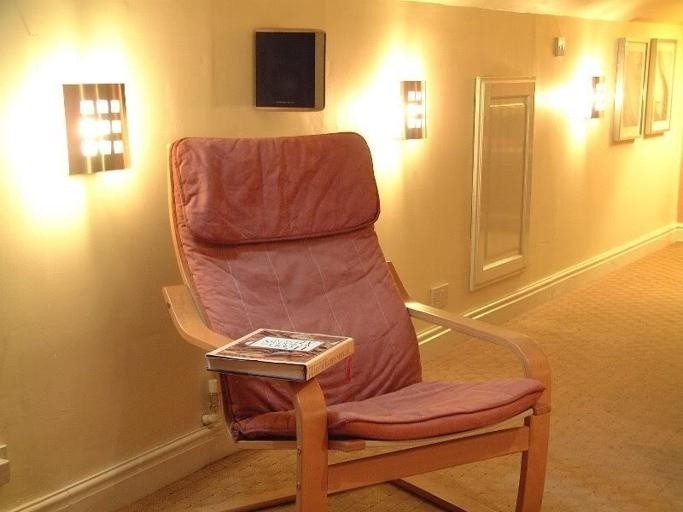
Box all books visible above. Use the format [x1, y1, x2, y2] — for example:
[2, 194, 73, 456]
[203, 328, 354, 382]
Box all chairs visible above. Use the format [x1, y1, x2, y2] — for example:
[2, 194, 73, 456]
[160, 127, 555, 512]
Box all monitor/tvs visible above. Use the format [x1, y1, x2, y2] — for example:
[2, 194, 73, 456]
[255, 28, 327, 113]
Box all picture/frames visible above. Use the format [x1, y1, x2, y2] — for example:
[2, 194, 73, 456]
[613, 35, 679, 141]
